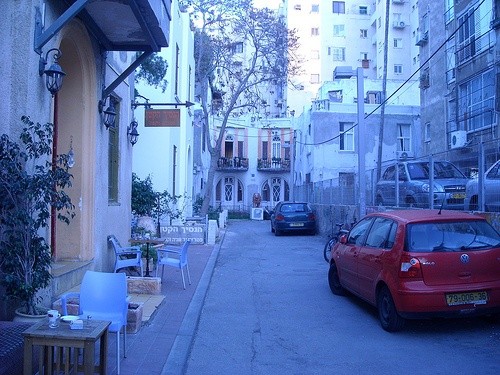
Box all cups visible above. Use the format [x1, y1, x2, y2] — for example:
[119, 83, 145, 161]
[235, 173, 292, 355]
[47, 310, 62, 328]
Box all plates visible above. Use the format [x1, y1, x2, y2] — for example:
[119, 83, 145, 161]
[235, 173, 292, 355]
[61, 315, 80, 322]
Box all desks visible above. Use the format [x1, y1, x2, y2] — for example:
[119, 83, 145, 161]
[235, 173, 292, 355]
[129, 239, 166, 277]
[20, 315, 113, 375]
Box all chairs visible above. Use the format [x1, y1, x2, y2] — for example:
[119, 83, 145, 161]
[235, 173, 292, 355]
[154, 240, 192, 290]
[61, 271, 129, 375]
[108, 235, 144, 278]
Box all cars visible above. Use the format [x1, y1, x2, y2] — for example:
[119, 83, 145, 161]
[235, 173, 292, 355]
[328, 209, 500, 334]
[267, 201, 317, 236]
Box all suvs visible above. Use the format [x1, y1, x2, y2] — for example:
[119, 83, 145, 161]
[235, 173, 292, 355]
[464, 159, 500, 213]
[373, 160, 471, 210]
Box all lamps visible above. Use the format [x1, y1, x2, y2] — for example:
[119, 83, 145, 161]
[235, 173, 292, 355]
[98, 94, 120, 130]
[39, 50, 65, 97]
[126, 121, 140, 147]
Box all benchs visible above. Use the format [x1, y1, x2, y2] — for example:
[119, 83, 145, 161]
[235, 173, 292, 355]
[0, 321, 40, 375]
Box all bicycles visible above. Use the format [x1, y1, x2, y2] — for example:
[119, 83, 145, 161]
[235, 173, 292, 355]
[323, 217, 358, 264]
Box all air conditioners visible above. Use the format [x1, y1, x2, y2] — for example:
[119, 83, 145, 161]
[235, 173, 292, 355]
[394, 21, 405, 29]
[451, 131, 467, 150]
[396, 151, 415, 160]
[415, 32, 425, 45]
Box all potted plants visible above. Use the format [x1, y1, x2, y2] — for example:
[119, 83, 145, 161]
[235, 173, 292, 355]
[0, 119, 76, 373]
